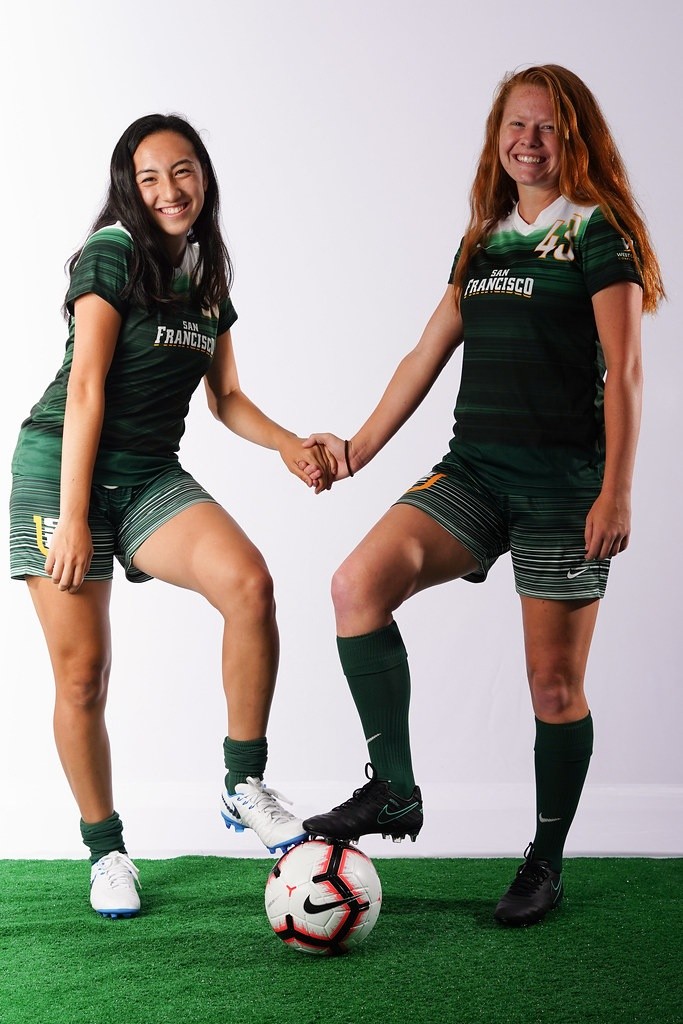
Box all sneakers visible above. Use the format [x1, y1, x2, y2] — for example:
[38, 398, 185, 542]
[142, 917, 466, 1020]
[86, 851, 140, 919]
[219, 777, 309, 855]
[494, 859, 564, 927]
[301, 779, 424, 844]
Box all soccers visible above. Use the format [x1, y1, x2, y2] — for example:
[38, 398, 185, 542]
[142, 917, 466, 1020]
[265, 839, 383, 958]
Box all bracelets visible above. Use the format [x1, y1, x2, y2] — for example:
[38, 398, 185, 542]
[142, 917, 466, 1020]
[344, 440, 354, 477]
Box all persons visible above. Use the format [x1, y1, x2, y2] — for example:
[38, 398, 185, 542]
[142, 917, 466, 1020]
[10, 114, 338, 918]
[298, 62, 666, 931]
